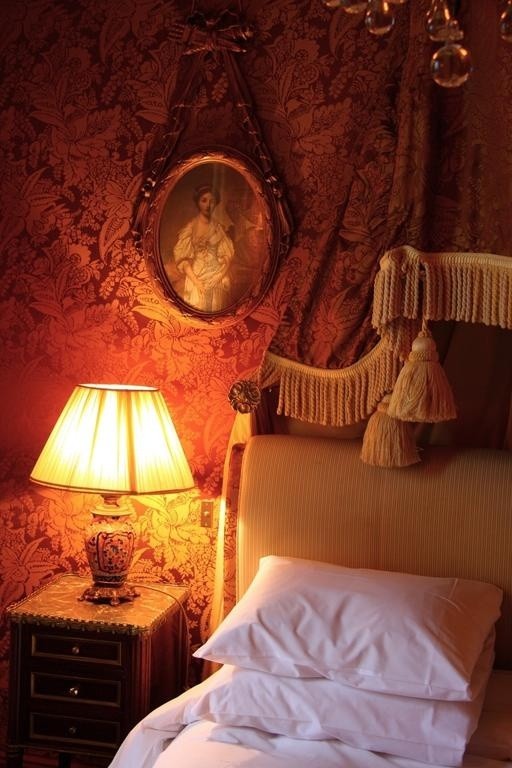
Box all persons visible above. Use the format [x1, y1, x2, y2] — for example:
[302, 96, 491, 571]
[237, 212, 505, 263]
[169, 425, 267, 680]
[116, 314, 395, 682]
[171, 184, 236, 313]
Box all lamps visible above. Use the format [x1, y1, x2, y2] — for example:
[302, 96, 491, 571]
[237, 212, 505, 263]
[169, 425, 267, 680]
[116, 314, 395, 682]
[21, 376, 203, 610]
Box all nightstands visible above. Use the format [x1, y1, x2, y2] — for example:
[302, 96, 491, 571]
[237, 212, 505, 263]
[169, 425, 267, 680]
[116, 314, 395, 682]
[1, 571, 196, 768]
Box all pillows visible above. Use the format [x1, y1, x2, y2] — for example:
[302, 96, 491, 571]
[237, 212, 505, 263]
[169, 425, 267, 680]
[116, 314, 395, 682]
[191, 548, 505, 701]
[188, 659, 495, 768]
[201, 718, 465, 768]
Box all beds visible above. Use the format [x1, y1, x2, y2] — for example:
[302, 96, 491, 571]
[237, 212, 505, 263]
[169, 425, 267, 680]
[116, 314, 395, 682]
[102, 233, 512, 766]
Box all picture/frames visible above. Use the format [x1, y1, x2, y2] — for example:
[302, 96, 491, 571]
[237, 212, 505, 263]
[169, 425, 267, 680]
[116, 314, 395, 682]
[128, 2, 302, 331]
